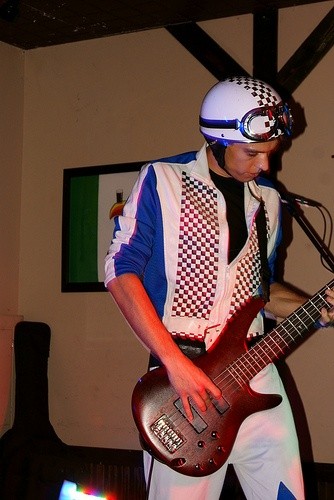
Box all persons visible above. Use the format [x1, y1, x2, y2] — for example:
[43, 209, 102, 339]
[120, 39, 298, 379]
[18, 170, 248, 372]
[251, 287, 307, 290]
[104, 77, 334, 500]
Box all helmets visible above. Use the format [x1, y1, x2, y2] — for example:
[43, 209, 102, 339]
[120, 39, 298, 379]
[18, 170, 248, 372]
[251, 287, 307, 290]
[199, 77, 288, 144]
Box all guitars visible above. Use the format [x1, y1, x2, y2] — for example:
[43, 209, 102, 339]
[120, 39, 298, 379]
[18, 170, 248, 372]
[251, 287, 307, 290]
[131, 278, 334, 478]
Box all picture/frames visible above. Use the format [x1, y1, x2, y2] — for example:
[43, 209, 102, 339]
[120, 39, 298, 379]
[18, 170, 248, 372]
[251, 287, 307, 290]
[61, 154, 175, 294]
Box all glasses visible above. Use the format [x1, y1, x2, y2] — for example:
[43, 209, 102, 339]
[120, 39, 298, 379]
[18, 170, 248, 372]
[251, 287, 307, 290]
[237, 103, 294, 142]
